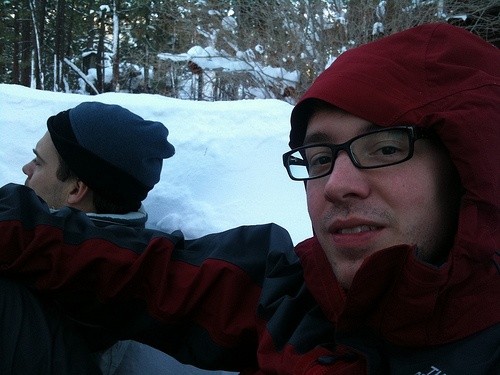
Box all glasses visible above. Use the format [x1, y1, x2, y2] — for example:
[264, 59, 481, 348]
[283, 125, 431, 181]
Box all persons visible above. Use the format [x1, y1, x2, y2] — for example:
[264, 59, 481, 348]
[0, 21, 500, 375]
[0, 100, 176, 375]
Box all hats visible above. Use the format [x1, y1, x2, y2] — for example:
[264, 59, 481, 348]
[47, 101, 176, 206]
